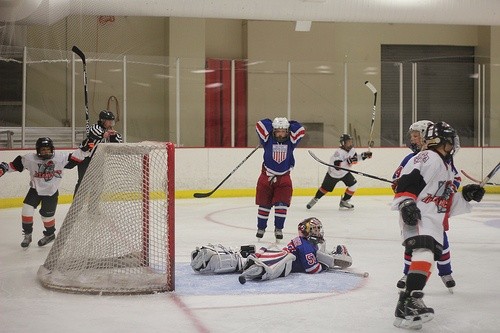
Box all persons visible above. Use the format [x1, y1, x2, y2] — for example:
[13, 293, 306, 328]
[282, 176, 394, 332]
[256, 117, 305, 243]
[73, 110, 122, 215]
[0, 137, 95, 251]
[392, 120, 456, 294]
[191, 217, 353, 285]
[306, 134, 372, 211]
[392, 122, 486, 329]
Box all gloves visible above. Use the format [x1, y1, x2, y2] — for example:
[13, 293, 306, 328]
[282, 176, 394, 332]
[347, 156, 358, 164]
[79, 138, 94, 153]
[462, 184, 485, 202]
[398, 199, 421, 226]
[361, 151, 372, 160]
[0, 162, 8, 177]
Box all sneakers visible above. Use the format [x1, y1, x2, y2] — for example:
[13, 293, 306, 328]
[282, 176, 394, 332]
[307, 198, 318, 209]
[339, 194, 354, 208]
[274, 228, 284, 242]
[21, 232, 32, 247]
[393, 290, 435, 330]
[38, 229, 55, 246]
[438, 273, 455, 294]
[397, 275, 408, 294]
[256, 228, 265, 242]
[239, 263, 263, 285]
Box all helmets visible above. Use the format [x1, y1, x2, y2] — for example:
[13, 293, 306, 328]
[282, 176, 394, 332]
[424, 121, 457, 152]
[99, 110, 115, 120]
[298, 217, 325, 244]
[410, 120, 435, 153]
[340, 135, 353, 149]
[272, 116, 290, 143]
[36, 138, 54, 159]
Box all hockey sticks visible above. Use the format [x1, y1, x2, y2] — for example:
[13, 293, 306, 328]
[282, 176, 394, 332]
[308, 149, 394, 184]
[329, 267, 369, 277]
[364, 81, 377, 152]
[480, 162, 500, 188]
[72, 45, 89, 136]
[194, 140, 264, 198]
[461, 170, 500, 186]
